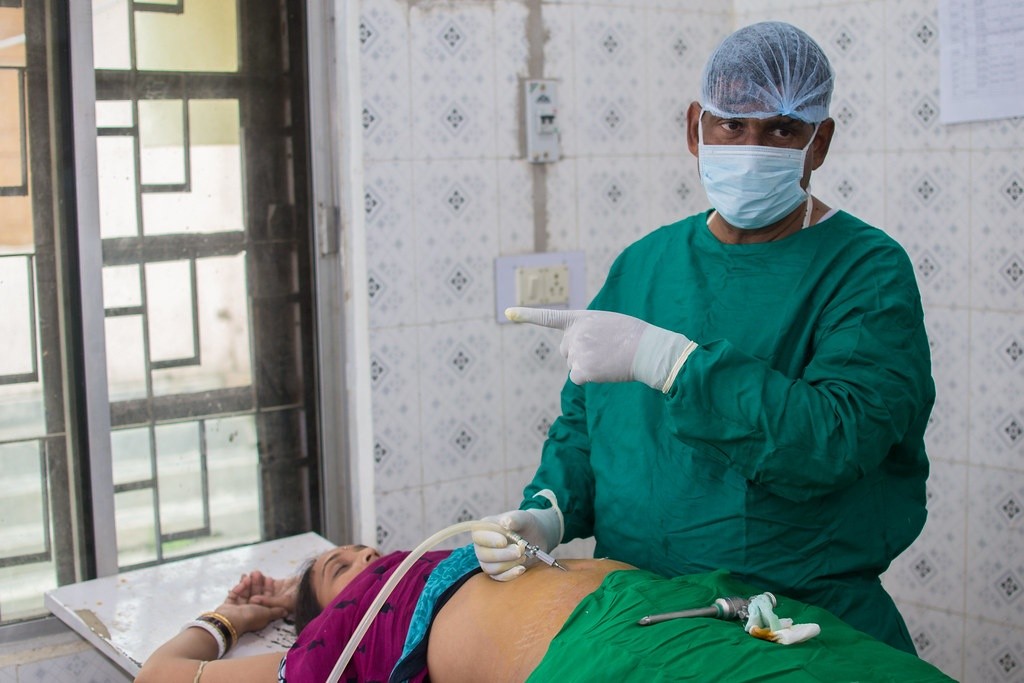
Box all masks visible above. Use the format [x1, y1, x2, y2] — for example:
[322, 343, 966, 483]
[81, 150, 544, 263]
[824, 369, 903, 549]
[698, 109, 821, 229]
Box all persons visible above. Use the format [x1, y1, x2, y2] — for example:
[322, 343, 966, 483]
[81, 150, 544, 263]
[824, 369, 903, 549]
[474, 23, 936, 660]
[135, 545, 962, 683]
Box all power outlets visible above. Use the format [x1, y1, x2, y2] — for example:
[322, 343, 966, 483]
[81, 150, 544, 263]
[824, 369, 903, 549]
[545, 267, 569, 304]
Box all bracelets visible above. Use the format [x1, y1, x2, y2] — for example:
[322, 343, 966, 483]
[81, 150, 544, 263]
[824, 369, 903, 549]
[182, 611, 238, 659]
